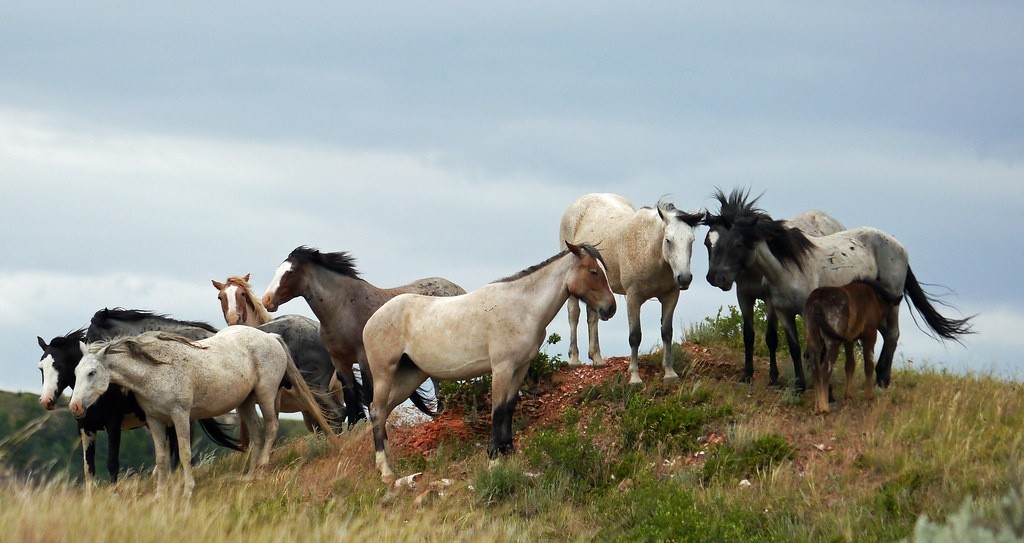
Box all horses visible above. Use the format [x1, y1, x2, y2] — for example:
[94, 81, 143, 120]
[559, 192, 707, 393]
[263, 246, 468, 434]
[699, 185, 980, 418]
[37, 273, 347, 506]
[361, 240, 618, 484]
